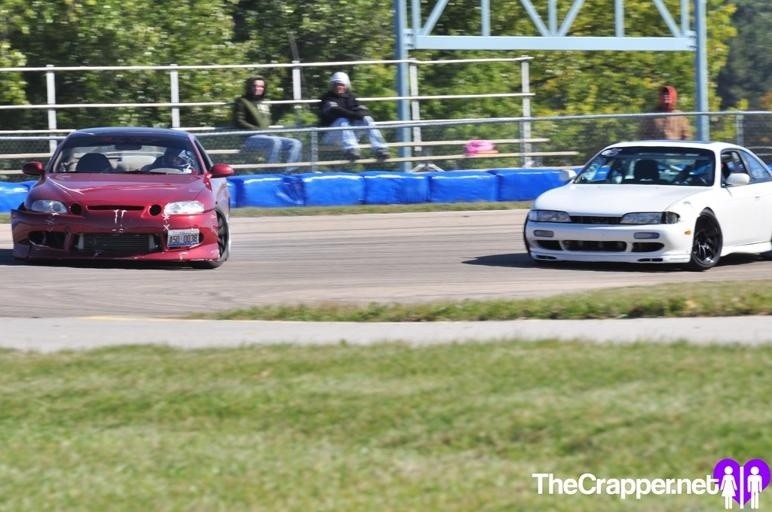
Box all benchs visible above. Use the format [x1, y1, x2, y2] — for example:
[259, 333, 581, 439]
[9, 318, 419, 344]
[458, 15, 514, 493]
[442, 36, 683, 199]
[240, 142, 351, 173]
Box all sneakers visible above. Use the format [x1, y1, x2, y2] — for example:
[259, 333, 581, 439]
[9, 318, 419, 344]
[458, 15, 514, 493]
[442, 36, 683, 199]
[345, 147, 361, 159]
[374, 147, 392, 159]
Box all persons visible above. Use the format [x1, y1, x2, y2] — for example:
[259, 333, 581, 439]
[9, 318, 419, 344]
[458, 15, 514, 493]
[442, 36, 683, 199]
[633, 85, 694, 141]
[235, 76, 302, 175]
[321, 71, 392, 161]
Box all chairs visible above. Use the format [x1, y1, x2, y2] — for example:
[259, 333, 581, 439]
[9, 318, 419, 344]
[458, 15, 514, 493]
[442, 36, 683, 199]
[630, 159, 661, 181]
[75, 153, 113, 173]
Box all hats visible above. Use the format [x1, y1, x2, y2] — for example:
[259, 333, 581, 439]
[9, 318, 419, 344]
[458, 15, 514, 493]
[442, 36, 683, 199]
[328, 71, 352, 88]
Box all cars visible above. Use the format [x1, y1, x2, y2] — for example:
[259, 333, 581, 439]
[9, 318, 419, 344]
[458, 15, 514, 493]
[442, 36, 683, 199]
[520, 135, 771, 276]
[8, 123, 237, 272]
